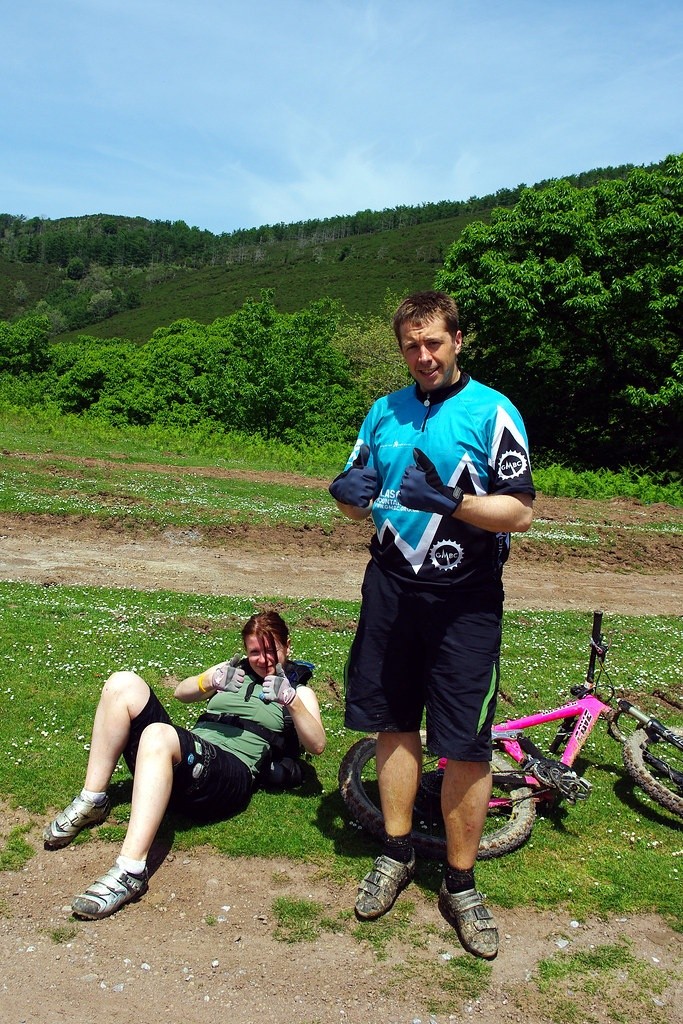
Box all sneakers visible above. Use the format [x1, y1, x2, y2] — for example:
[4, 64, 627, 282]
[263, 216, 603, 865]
[437, 879, 500, 959]
[355, 848, 416, 918]
[71, 862, 150, 921]
[39, 795, 108, 846]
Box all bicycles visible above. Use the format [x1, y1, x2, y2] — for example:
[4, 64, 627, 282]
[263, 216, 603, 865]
[338, 611, 683, 860]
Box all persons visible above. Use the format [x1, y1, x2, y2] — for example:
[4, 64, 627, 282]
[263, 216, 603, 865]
[43, 610, 327, 920]
[328, 291, 536, 959]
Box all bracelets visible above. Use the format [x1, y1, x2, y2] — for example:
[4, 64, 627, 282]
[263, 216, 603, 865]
[198, 675, 207, 694]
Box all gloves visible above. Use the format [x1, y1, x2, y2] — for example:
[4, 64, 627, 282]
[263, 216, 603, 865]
[328, 444, 378, 508]
[396, 449, 464, 518]
[259, 663, 297, 707]
[197, 653, 245, 693]
